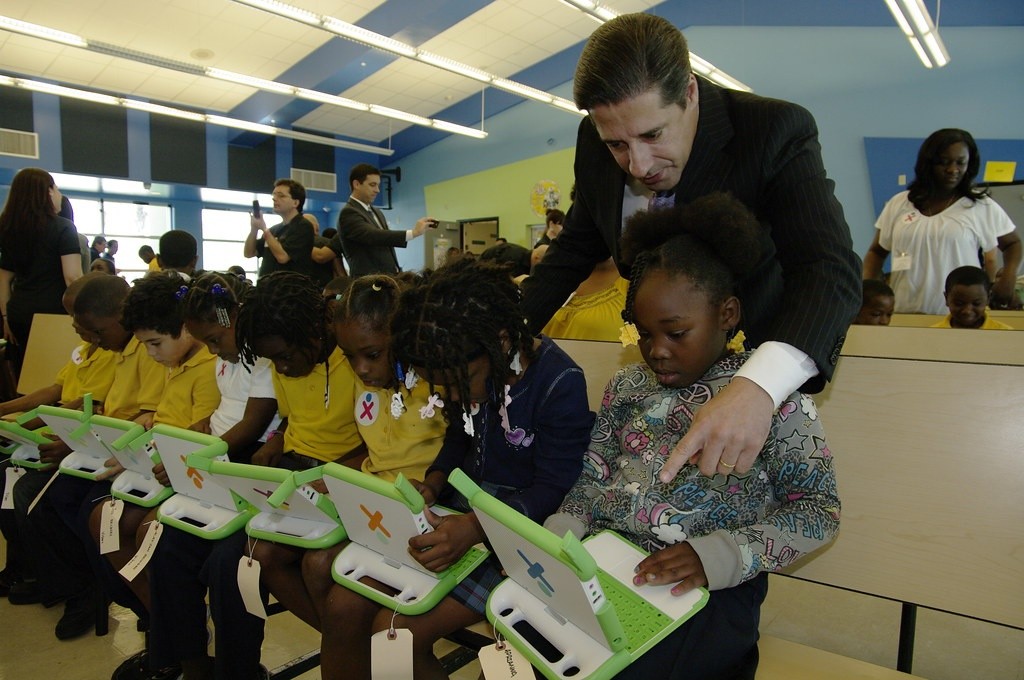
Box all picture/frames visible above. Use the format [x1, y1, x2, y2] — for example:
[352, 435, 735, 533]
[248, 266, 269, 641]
[526, 223, 547, 250]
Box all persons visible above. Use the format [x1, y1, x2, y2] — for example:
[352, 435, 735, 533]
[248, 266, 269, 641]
[442, 208, 748, 355]
[147, 276, 368, 680]
[494, 190, 842, 679]
[926, 266, 1013, 330]
[852, 279, 894, 327]
[1, 168, 341, 401]
[7, 269, 589, 679]
[861, 128, 1024, 311]
[337, 163, 439, 281]
[518, 15, 864, 484]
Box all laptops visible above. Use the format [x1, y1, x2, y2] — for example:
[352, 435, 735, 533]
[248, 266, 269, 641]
[0, 392, 709, 680]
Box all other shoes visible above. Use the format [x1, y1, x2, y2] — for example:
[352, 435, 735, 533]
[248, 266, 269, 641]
[55, 591, 113, 640]
[0, 565, 43, 605]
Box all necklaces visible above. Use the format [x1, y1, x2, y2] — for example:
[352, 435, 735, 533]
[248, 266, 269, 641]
[928, 192, 956, 216]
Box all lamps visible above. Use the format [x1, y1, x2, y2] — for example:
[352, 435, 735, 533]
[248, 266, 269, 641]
[883, 0, 952, 72]
[0, 0, 754, 157]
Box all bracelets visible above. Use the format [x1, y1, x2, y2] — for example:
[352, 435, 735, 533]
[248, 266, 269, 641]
[3, 316, 7, 321]
[2, 314, 7, 316]
[262, 229, 270, 239]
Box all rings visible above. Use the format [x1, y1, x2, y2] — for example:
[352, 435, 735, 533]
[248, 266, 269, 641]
[720, 459, 736, 468]
[1000, 304, 1008, 308]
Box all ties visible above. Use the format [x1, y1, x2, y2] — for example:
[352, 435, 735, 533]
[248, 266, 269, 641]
[367, 210, 381, 230]
[646, 188, 675, 212]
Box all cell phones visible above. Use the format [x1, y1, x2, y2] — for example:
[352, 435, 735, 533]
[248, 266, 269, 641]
[252, 200, 260, 219]
[428, 220, 439, 229]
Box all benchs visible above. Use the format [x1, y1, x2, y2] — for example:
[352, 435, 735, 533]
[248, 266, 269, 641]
[548, 341, 1024, 680]
